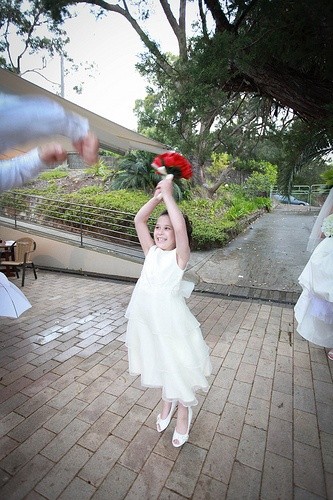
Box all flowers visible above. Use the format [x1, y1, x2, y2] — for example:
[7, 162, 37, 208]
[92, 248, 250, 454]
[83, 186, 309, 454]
[152, 152, 192, 200]
[321, 214, 333, 238]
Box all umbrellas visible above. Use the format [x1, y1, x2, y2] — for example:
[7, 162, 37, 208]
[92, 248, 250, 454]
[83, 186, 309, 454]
[0, 272, 33, 318]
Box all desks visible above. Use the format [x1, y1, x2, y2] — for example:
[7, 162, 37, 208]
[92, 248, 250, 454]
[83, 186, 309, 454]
[0, 241, 20, 277]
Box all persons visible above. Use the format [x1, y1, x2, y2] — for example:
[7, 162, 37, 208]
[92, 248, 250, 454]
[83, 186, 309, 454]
[122, 179, 213, 447]
[293, 185, 333, 361]
[0, 92, 100, 191]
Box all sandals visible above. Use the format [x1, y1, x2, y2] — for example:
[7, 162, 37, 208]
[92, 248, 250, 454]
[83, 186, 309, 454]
[156, 401, 192, 447]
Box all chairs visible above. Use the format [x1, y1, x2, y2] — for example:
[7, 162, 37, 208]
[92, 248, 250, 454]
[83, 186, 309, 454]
[0, 237, 37, 287]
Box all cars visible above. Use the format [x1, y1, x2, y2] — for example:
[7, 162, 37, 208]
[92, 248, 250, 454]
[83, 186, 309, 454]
[273, 195, 310, 206]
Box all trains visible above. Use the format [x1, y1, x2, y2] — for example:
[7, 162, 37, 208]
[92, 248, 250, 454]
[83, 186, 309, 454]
[272, 184, 330, 195]
